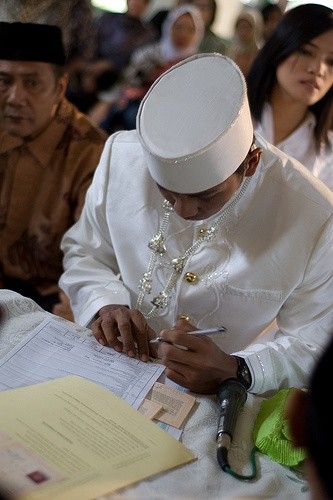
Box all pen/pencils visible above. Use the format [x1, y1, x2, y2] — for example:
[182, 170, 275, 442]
[150, 326, 226, 344]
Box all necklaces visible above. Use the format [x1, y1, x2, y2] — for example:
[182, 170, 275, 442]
[136, 174, 254, 322]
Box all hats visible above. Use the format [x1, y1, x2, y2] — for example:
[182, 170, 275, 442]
[0, 21, 66, 68]
[136, 52, 254, 194]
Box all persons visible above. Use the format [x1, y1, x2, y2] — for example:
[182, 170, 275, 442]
[65, 0, 282, 137]
[0, 22, 110, 325]
[246, 4, 333, 191]
[59, 49, 333, 397]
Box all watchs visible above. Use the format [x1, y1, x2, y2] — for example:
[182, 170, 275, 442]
[235, 356, 252, 390]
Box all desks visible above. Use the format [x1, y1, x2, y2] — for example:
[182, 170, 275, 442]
[0, 289, 311, 500]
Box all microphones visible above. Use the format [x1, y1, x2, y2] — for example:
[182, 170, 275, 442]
[216, 377, 248, 469]
